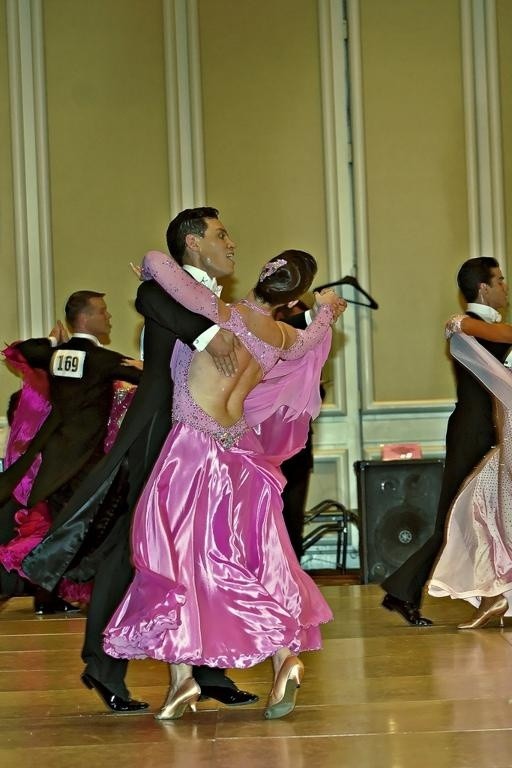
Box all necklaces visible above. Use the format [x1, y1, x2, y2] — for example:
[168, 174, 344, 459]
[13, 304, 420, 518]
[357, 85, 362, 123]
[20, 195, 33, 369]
[238, 300, 273, 316]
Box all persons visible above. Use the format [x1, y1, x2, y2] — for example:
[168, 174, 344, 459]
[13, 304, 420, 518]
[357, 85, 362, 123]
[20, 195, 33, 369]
[429, 313, 511, 630]
[102, 245, 341, 725]
[281, 373, 327, 565]
[381, 257, 509, 625]
[0, 291, 141, 615]
[23, 207, 347, 712]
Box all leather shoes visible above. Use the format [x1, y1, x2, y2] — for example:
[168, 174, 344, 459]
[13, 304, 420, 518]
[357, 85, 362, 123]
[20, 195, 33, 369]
[33, 598, 80, 616]
[77, 674, 149, 713]
[380, 593, 433, 629]
[196, 680, 258, 707]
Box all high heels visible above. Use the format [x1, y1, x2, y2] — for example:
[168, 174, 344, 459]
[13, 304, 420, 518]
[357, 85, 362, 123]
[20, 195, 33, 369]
[454, 596, 510, 632]
[262, 656, 307, 721]
[151, 676, 204, 722]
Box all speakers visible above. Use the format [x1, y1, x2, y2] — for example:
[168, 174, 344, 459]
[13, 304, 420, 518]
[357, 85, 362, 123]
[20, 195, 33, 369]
[354, 459, 447, 586]
